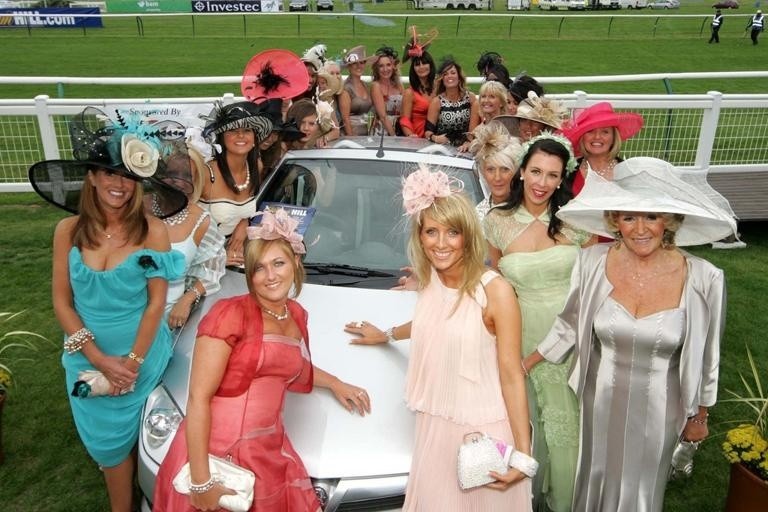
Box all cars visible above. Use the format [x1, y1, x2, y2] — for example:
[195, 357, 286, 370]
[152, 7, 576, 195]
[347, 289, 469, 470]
[316, 0, 334, 12]
[289, 0, 308, 12]
[711, 0, 739, 9]
[647, 0, 680, 10]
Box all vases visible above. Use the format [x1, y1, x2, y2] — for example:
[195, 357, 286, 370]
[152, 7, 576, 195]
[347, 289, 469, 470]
[723, 463, 767, 512]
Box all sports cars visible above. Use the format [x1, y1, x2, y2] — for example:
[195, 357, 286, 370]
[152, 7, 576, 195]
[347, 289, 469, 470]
[130, 127, 497, 509]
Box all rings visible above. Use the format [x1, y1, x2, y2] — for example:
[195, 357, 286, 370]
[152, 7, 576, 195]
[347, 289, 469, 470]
[357, 391, 363, 397]
[356, 320, 364, 328]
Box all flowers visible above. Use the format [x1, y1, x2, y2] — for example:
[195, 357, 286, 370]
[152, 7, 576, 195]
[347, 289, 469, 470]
[720, 421, 767, 481]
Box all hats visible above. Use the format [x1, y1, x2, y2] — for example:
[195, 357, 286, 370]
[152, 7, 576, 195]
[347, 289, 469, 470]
[164, 142, 205, 204]
[554, 156, 738, 249]
[165, 124, 213, 165]
[202, 101, 274, 146]
[241, 44, 375, 103]
[27, 127, 190, 220]
[478, 51, 560, 129]
[563, 100, 644, 157]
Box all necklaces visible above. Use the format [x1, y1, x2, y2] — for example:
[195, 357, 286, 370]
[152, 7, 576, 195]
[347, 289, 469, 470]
[263, 305, 288, 320]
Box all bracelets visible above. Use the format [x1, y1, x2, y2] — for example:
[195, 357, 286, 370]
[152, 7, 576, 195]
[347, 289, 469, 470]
[510, 451, 539, 478]
[387, 328, 398, 342]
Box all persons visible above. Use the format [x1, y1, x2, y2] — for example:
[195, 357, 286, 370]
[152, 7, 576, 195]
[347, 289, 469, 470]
[154, 207, 371, 512]
[710, 10, 724, 43]
[27, 101, 275, 512]
[464, 49, 738, 512]
[243, 26, 479, 204]
[345, 142, 533, 512]
[745, 9, 765, 45]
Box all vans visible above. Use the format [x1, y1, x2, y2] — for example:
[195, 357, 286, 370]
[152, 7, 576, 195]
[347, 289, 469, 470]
[506, 0, 530, 12]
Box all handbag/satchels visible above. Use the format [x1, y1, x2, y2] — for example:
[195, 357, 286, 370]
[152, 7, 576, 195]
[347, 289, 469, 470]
[670, 438, 704, 478]
[171, 452, 257, 512]
[455, 431, 508, 492]
